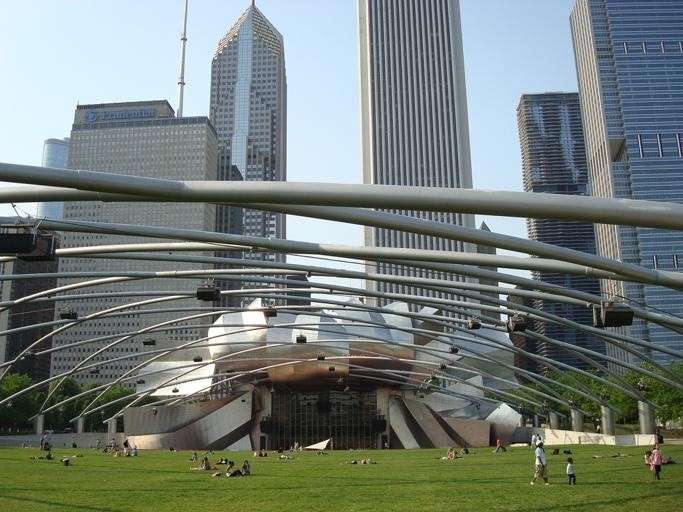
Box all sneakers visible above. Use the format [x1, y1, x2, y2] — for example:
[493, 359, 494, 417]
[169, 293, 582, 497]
[655, 474, 660, 480]
[530, 480, 550, 486]
[568, 482, 576, 485]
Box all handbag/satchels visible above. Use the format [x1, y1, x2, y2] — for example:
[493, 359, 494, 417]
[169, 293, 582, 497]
[650, 465, 655, 471]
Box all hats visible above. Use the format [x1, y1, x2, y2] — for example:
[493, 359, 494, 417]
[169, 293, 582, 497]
[535, 440, 542, 447]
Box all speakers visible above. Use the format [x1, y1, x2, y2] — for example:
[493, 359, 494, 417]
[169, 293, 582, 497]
[412, 300, 635, 397]
[193, 282, 351, 403]
[0, 221, 57, 263]
[21, 304, 180, 393]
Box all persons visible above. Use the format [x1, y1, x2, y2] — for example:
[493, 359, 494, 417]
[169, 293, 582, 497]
[566, 458, 576, 485]
[530, 440, 550, 486]
[279, 455, 293, 460]
[491, 438, 507, 453]
[258, 450, 267, 457]
[40, 432, 51, 451]
[531, 432, 542, 449]
[293, 441, 300, 452]
[60, 459, 69, 466]
[278, 447, 284, 453]
[96, 439, 100, 449]
[190, 457, 210, 471]
[189, 452, 197, 462]
[45, 452, 51, 460]
[216, 457, 250, 477]
[106, 437, 138, 457]
[384, 441, 388, 449]
[346, 458, 376, 465]
[169, 446, 174, 453]
[644, 443, 665, 480]
[447, 445, 469, 460]
